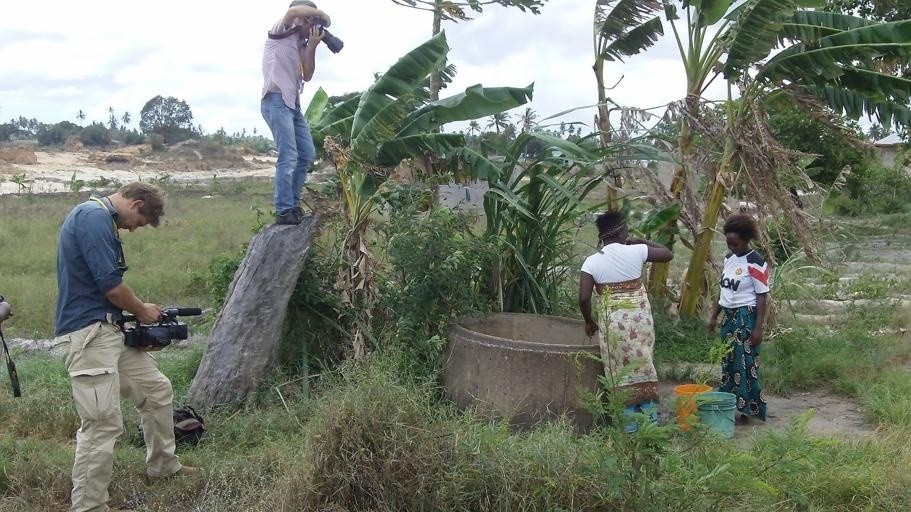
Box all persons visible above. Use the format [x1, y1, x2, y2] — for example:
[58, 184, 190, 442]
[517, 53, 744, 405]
[579, 208, 674, 432]
[260, 0, 332, 223]
[52, 180, 200, 510]
[708, 213, 769, 425]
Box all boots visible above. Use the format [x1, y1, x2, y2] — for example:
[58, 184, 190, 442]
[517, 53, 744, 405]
[626, 403, 657, 433]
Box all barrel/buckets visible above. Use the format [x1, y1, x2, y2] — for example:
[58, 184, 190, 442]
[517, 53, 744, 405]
[692, 392, 737, 441]
[673, 383, 712, 432]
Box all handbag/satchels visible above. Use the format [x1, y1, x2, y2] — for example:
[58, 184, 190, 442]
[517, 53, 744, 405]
[173, 406, 205, 444]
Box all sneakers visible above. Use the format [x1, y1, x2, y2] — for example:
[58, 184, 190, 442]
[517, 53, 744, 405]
[148, 465, 193, 482]
[276, 207, 312, 225]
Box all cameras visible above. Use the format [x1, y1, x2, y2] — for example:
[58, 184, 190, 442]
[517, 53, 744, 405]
[306, 19, 345, 53]
